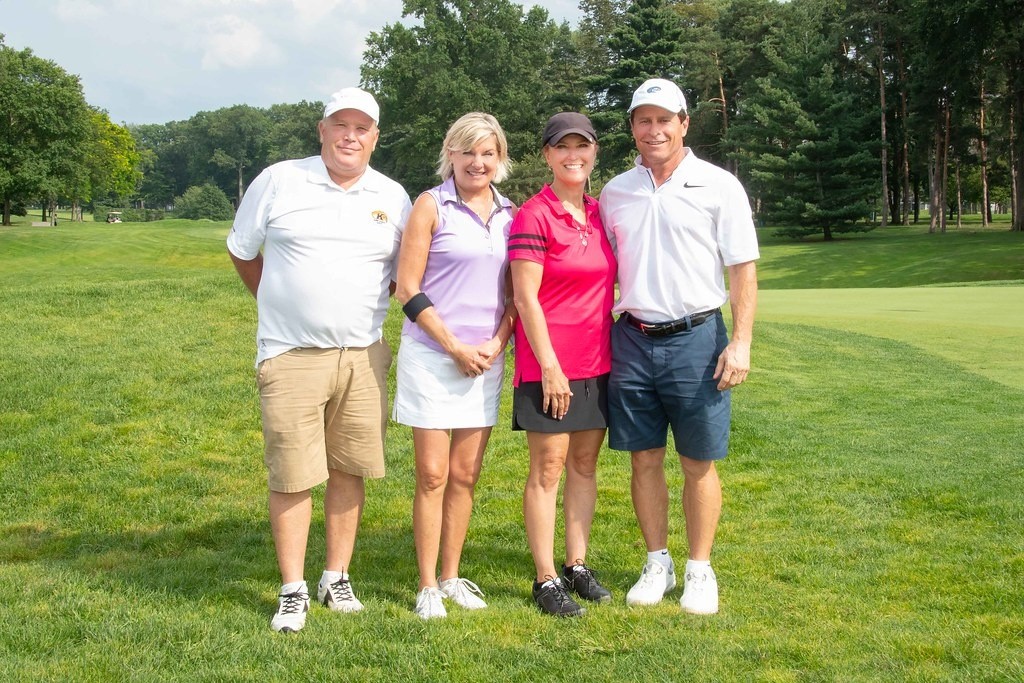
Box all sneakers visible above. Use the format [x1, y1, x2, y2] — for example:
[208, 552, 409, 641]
[626, 557, 677, 606]
[681, 562, 718, 614]
[317, 567, 365, 612]
[532, 575, 586, 617]
[271, 583, 311, 632]
[412, 583, 448, 619]
[437, 575, 486, 609]
[561, 559, 611, 604]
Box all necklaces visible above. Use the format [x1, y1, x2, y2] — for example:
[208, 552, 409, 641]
[554, 186, 588, 247]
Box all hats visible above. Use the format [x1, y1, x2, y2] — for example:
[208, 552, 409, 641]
[543, 111, 598, 147]
[323, 87, 380, 126]
[628, 79, 688, 121]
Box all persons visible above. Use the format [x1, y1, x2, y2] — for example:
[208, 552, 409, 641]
[54, 214, 58, 225]
[598, 78, 759, 615]
[227, 86, 412, 633]
[509, 113, 618, 618]
[395, 111, 518, 619]
[107, 214, 119, 223]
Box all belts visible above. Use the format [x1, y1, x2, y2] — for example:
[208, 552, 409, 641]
[619, 309, 720, 339]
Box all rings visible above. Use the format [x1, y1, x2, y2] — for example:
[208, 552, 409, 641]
[468, 371, 473, 375]
